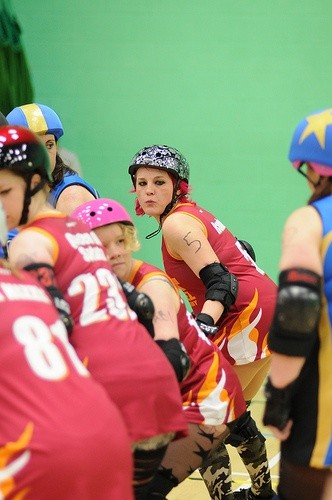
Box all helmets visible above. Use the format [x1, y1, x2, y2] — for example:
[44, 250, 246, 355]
[288, 107, 332, 179]
[5, 102, 65, 141]
[128, 144, 191, 185]
[0, 124, 55, 184]
[68, 198, 135, 233]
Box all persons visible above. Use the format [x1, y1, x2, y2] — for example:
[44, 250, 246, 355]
[0, 100, 245, 500]
[128, 145, 277, 500]
[262, 109, 332, 500]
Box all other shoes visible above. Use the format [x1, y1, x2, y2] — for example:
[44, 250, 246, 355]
[222, 485, 281, 500]
